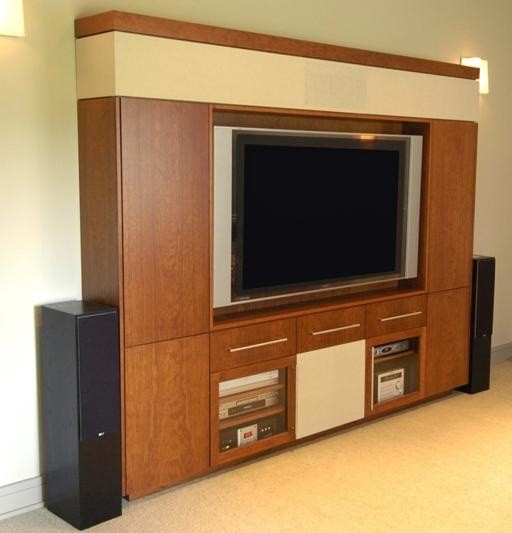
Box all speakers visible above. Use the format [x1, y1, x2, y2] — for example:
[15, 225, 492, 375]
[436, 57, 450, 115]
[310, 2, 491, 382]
[35, 300, 121, 530]
[454, 255, 495, 394]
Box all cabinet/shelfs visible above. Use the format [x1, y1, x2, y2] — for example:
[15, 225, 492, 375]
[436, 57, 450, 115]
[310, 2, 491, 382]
[296, 301, 365, 445]
[77, 95, 209, 348]
[366, 293, 426, 423]
[121, 333, 210, 502]
[398, 117, 479, 292]
[210, 310, 295, 476]
[424, 283, 472, 403]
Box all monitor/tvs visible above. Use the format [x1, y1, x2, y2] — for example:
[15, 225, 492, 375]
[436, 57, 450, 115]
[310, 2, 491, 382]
[213, 125, 424, 309]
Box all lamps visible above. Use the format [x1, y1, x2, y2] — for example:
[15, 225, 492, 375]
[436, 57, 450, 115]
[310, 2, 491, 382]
[1, 0, 26, 38]
[462, 57, 489, 94]
